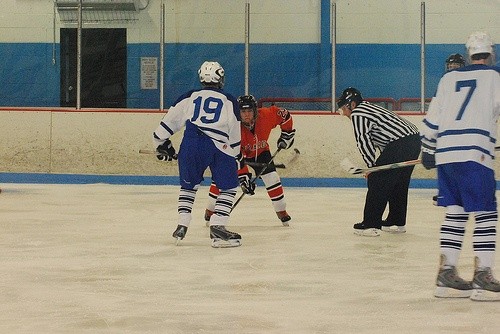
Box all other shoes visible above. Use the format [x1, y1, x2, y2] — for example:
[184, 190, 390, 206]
[433, 195, 438, 205]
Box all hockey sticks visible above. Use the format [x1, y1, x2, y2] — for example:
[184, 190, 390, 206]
[340, 147, 500, 175]
[230, 146, 281, 212]
[139, 147, 301, 170]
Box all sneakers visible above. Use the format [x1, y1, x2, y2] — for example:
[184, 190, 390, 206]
[172, 225, 188, 245]
[434, 254, 473, 298]
[210, 225, 242, 247]
[471, 256, 500, 300]
[276, 211, 291, 226]
[381, 219, 406, 232]
[205, 209, 213, 226]
[353, 222, 381, 236]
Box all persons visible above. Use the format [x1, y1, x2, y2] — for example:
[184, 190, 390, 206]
[337, 87, 422, 230]
[153, 61, 245, 241]
[205, 95, 294, 222]
[422, 32, 500, 294]
[433, 54, 465, 201]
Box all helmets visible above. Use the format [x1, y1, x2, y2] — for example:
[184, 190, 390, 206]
[337, 88, 363, 109]
[236, 95, 257, 119]
[446, 53, 465, 63]
[469, 32, 492, 56]
[197, 61, 224, 84]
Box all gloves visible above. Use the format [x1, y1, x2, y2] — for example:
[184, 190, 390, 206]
[277, 129, 296, 149]
[235, 151, 245, 170]
[238, 173, 255, 194]
[422, 152, 436, 170]
[152, 139, 177, 161]
